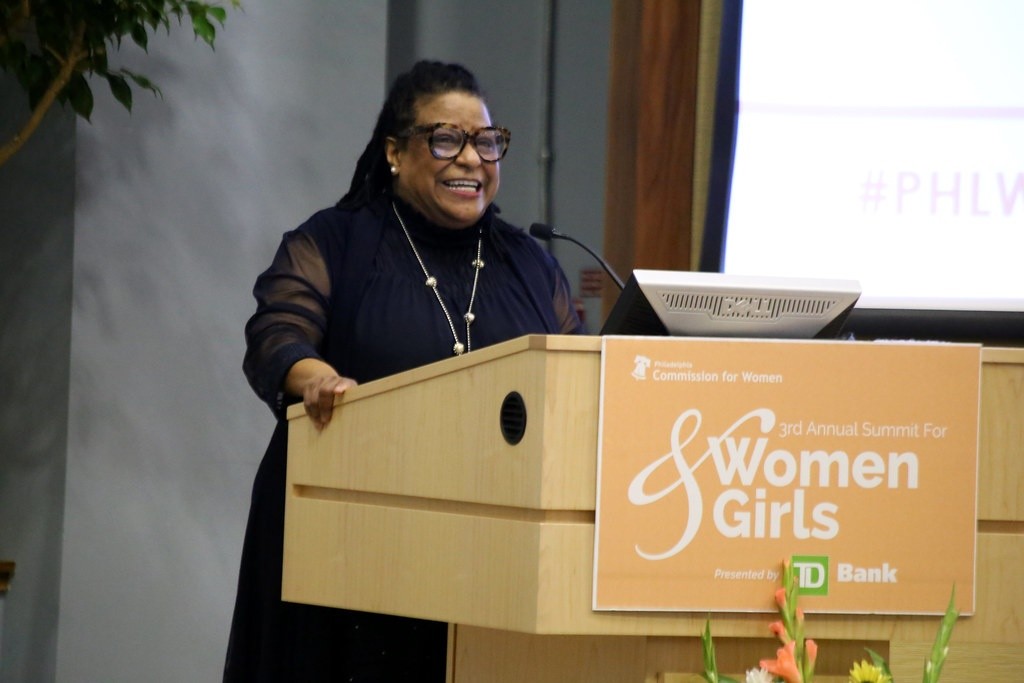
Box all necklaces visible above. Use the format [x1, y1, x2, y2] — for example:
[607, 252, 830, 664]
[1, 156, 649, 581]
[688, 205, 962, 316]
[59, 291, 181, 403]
[392, 190, 484, 359]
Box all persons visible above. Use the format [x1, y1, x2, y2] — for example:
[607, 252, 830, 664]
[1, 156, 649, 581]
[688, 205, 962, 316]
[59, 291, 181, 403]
[216, 58, 591, 683]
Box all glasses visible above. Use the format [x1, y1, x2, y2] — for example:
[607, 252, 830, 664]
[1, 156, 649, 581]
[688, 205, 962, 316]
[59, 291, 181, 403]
[394, 123, 512, 164]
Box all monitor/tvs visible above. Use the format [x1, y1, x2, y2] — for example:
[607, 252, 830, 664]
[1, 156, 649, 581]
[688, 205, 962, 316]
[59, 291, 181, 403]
[599, 268, 862, 340]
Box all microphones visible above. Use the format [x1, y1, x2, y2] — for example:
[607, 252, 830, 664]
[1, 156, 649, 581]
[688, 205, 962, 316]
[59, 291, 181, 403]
[529, 223, 625, 291]
